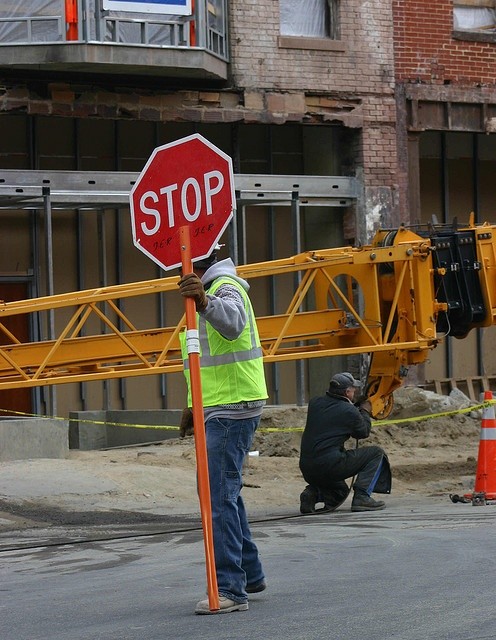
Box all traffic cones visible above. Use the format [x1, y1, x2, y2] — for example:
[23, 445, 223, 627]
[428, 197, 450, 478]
[464, 391, 495, 500]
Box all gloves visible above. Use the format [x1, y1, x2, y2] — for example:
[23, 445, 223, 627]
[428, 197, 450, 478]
[359, 400, 372, 413]
[178, 272, 208, 310]
[179, 407, 195, 440]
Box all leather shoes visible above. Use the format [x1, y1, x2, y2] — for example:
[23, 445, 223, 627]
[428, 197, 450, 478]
[300, 491, 316, 515]
[194, 594, 249, 614]
[351, 489, 385, 511]
[244, 579, 265, 593]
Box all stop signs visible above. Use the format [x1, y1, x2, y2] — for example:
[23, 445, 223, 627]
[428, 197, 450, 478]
[130, 133, 236, 271]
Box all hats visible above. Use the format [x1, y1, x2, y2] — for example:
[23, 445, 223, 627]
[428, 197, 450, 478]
[330, 373, 363, 389]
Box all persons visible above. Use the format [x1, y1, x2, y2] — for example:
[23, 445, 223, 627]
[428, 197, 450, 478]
[178, 242, 269, 615]
[299, 372, 392, 514]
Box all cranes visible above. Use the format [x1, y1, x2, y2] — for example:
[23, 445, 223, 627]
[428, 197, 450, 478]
[0, 212, 495, 420]
